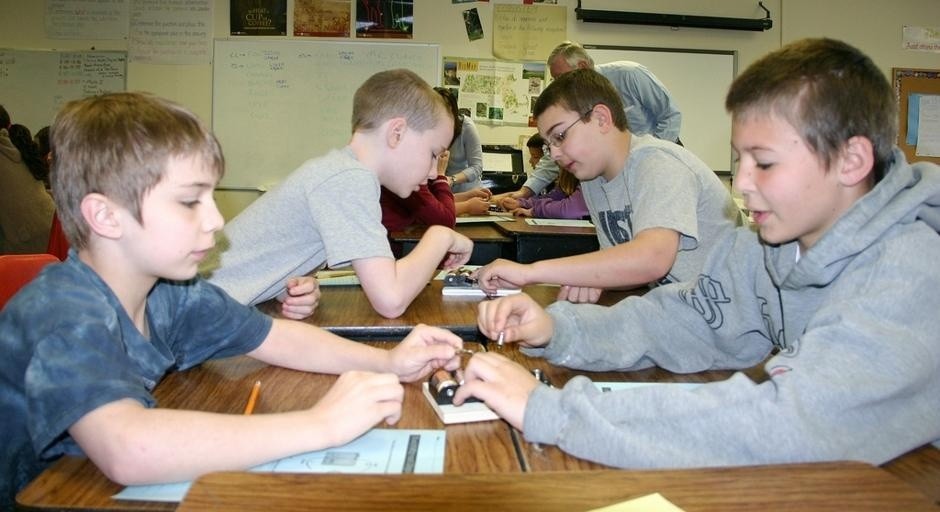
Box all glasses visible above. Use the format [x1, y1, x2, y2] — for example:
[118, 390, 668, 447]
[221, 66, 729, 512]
[541, 109, 592, 158]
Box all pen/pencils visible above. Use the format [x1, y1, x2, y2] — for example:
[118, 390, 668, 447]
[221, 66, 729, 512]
[313, 271, 357, 279]
[243, 381, 262, 416]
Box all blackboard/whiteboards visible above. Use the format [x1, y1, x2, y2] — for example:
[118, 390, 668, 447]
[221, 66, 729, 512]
[212, 35, 443, 190]
[583, 45, 740, 175]
[1, 49, 129, 140]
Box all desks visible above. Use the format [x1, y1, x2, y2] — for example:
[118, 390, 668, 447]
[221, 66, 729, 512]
[175, 462, 939, 512]
[517, 275, 659, 310]
[391, 221, 515, 266]
[489, 340, 935, 472]
[16, 343, 526, 509]
[494, 210, 600, 242]
[253, 266, 491, 342]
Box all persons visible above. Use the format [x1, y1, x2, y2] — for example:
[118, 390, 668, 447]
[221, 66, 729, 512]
[499, 133, 600, 261]
[467, 66, 744, 304]
[195, 68, 475, 320]
[1, 91, 463, 512]
[451, 36, 940, 471]
[434, 87, 483, 193]
[0, 105, 57, 256]
[378, 151, 492, 231]
[510, 41, 682, 198]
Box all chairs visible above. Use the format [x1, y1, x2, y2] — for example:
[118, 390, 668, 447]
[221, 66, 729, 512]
[48, 207, 74, 264]
[2, 253, 61, 306]
